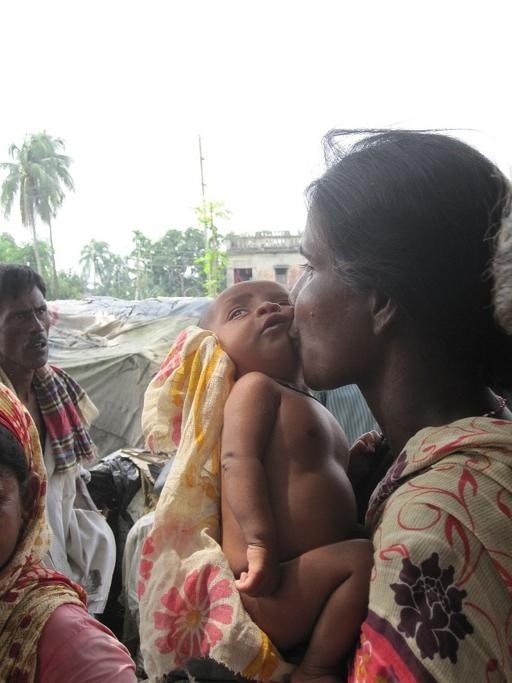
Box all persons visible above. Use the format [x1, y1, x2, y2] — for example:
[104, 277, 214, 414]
[1, 385, 139, 682]
[200, 279, 380, 683]
[2, 265, 134, 640]
[290, 129, 512, 683]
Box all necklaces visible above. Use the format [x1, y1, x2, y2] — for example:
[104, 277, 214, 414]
[275, 379, 322, 403]
